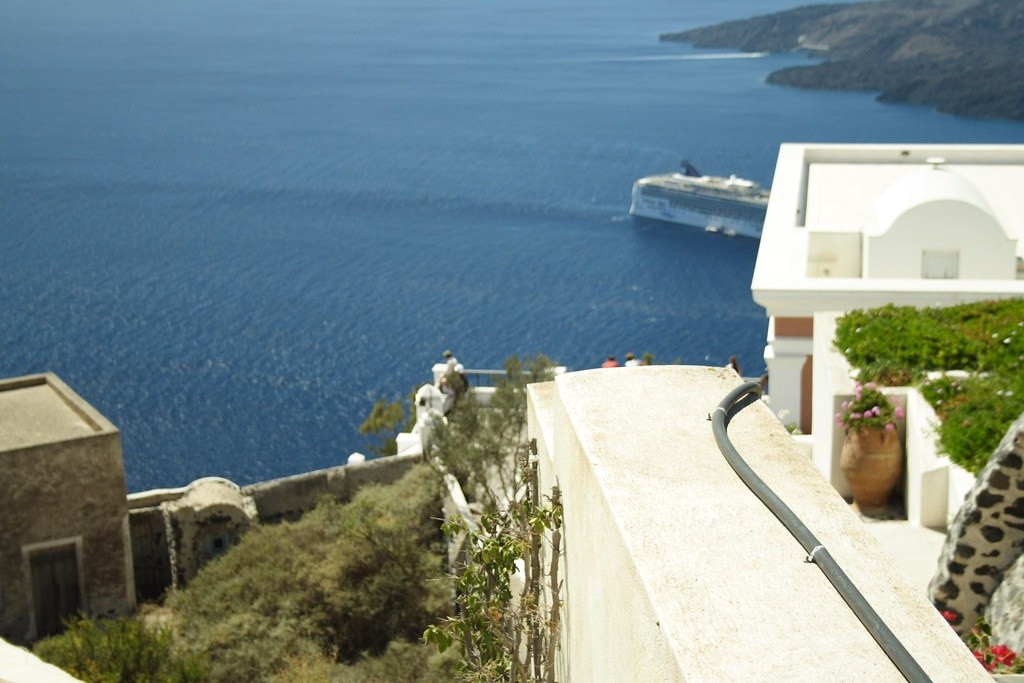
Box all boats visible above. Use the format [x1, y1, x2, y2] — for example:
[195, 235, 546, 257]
[627, 159, 773, 240]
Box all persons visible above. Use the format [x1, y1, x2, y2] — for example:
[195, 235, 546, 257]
[443, 350, 458, 374]
[726, 355, 742, 376]
[602, 356, 619, 368]
[624, 352, 647, 366]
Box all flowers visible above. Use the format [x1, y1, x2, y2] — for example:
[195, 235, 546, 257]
[835, 377, 905, 434]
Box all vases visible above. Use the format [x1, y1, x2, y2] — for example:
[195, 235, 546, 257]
[839, 424, 904, 515]
